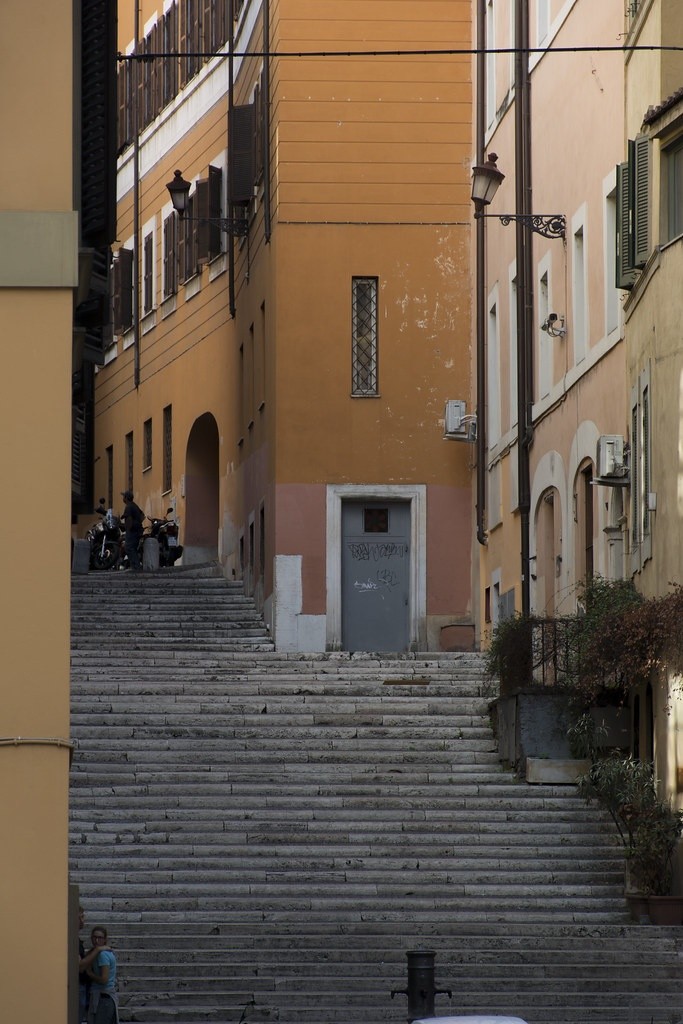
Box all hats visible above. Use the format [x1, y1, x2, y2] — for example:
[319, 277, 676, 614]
[120, 491, 134, 499]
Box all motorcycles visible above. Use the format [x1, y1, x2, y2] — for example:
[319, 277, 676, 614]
[144, 505, 182, 568]
[82, 499, 127, 572]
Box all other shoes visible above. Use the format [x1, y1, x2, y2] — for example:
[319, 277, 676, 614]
[125, 567, 140, 571]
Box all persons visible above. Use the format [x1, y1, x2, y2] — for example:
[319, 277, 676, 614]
[81, 926, 118, 1024]
[117, 491, 145, 571]
[72, 935, 114, 1024]
